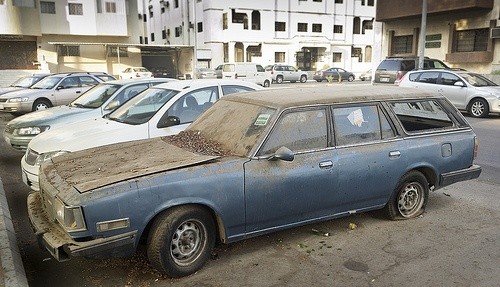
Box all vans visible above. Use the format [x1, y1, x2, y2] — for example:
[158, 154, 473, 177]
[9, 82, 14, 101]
[222, 62, 273, 87]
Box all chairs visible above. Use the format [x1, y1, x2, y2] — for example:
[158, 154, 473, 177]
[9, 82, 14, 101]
[183, 95, 200, 114]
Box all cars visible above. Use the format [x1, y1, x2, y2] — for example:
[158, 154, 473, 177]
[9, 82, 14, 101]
[196, 68, 217, 79]
[26, 83, 482, 279]
[0, 74, 53, 96]
[149, 67, 171, 78]
[215, 64, 224, 78]
[2, 77, 218, 154]
[118, 66, 154, 80]
[21, 78, 270, 192]
[359, 69, 373, 81]
[312, 67, 355, 82]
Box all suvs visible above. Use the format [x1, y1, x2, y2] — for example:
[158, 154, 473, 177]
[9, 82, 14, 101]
[264, 64, 308, 84]
[0, 71, 116, 116]
[370, 55, 449, 85]
[397, 68, 500, 119]
